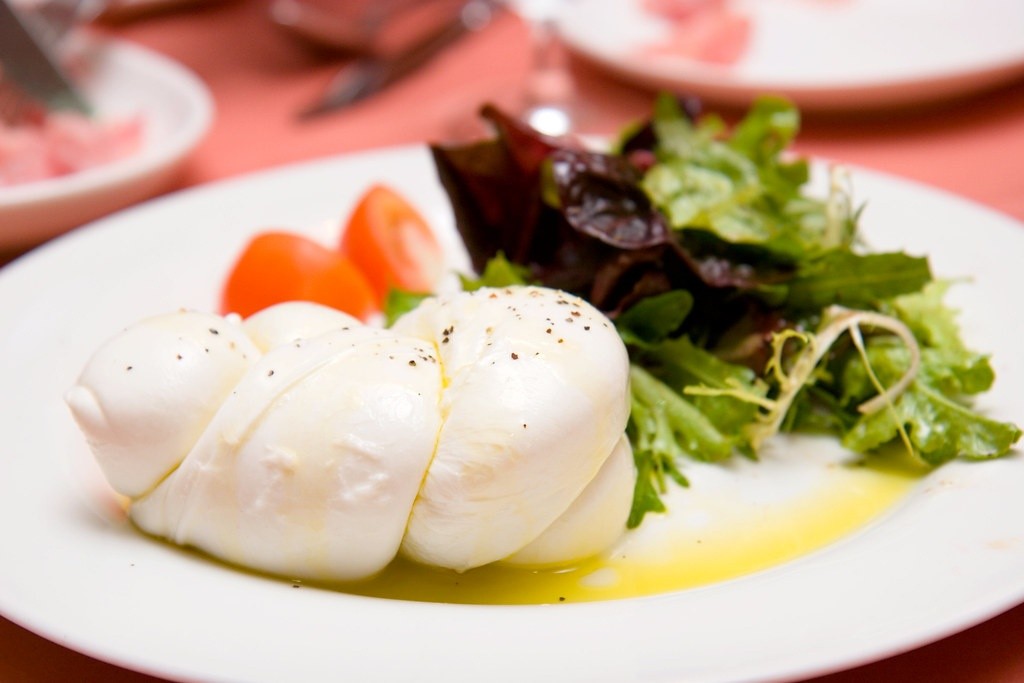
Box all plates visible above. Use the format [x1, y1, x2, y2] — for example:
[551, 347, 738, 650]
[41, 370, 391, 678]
[3, 137, 1024, 683]
[539, 4, 1022, 120]
[1, 19, 224, 259]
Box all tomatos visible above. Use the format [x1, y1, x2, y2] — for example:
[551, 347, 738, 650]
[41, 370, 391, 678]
[220, 182, 438, 321]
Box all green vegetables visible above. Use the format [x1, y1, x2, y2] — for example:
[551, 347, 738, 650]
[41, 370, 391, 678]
[452, 98, 1021, 532]
